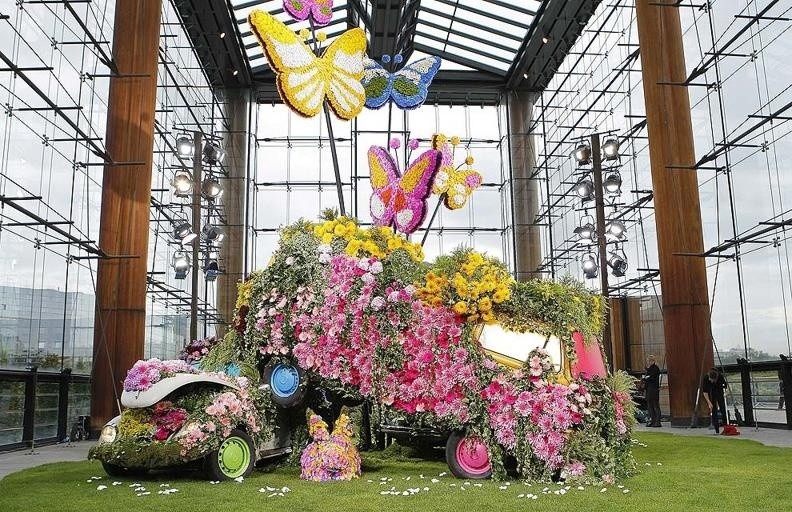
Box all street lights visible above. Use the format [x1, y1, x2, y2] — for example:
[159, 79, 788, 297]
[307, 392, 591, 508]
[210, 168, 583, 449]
[570, 129, 628, 376]
[172, 128, 224, 343]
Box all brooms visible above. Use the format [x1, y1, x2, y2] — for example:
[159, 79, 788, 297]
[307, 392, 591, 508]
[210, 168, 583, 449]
[708, 409, 717, 435]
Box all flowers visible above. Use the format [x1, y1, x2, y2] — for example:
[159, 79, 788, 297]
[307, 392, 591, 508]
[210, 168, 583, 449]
[118, 215, 641, 491]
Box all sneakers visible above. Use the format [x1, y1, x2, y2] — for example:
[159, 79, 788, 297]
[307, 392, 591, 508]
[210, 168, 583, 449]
[645, 422, 663, 427]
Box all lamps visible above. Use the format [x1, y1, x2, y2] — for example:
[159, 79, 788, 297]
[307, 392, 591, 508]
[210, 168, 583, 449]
[570, 134, 627, 212]
[163, 128, 230, 206]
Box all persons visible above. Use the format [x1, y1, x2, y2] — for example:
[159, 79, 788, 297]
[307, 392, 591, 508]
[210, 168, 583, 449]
[701, 368, 728, 436]
[640, 354, 663, 428]
